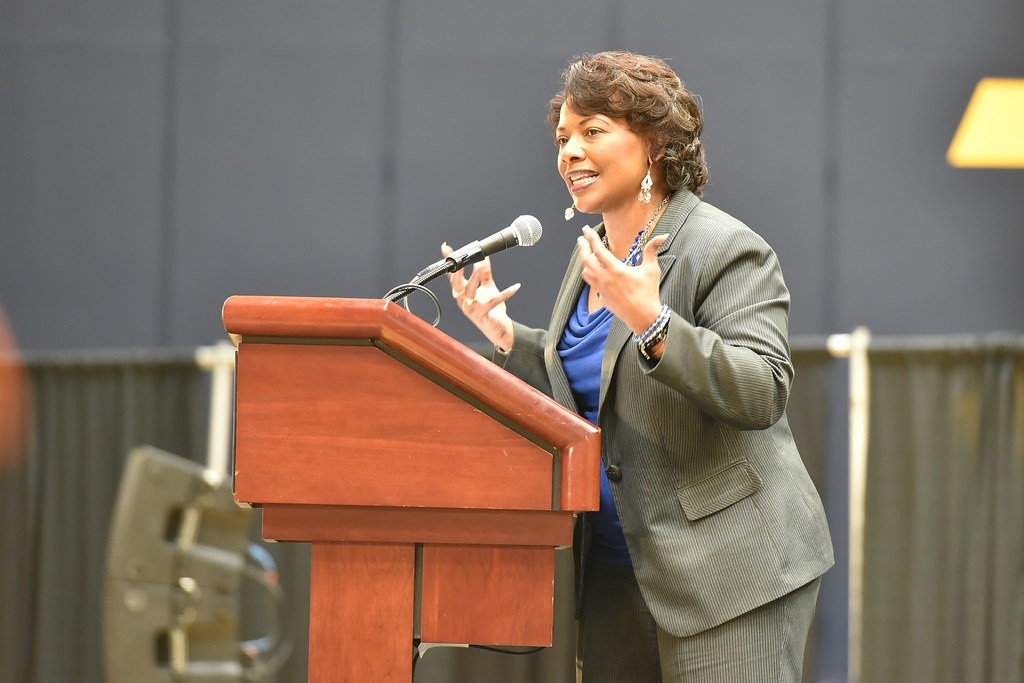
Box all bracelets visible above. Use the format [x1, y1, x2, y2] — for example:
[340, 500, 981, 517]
[493, 341, 512, 358]
[629, 303, 672, 362]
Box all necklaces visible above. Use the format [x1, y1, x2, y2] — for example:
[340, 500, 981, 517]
[594, 190, 674, 299]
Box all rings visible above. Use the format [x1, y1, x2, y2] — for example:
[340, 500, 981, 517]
[450, 286, 466, 298]
[459, 293, 475, 305]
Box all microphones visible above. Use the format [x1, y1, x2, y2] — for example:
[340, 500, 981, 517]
[445, 215, 542, 273]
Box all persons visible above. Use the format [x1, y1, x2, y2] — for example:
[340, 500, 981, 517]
[439, 49, 837, 683]
